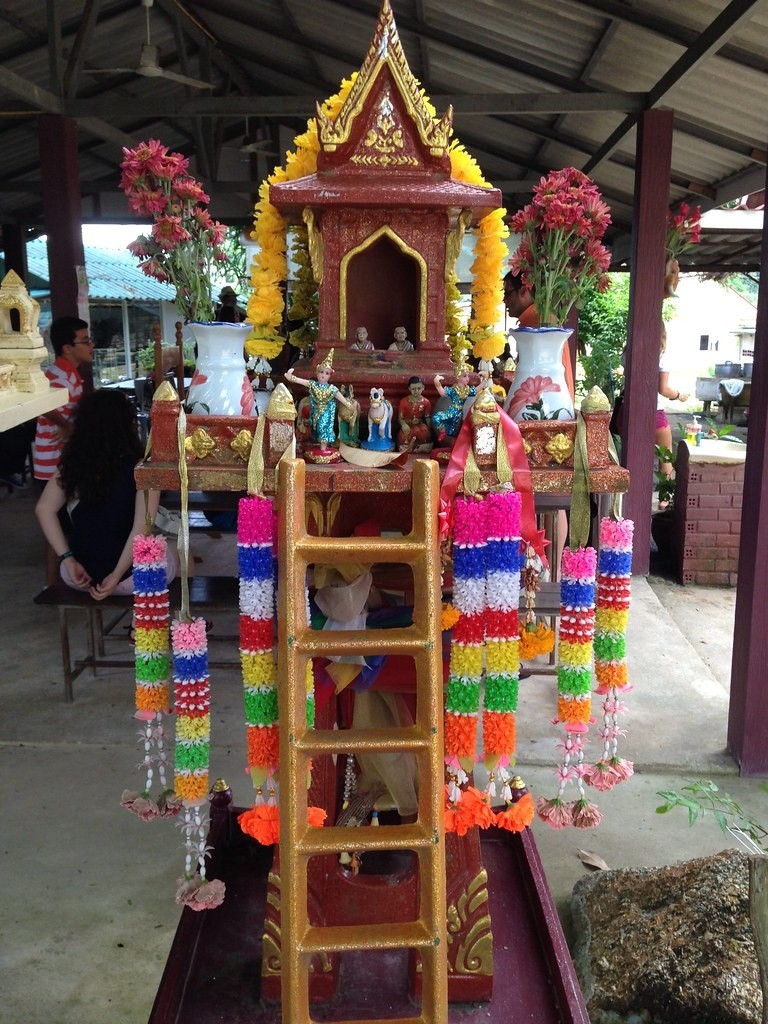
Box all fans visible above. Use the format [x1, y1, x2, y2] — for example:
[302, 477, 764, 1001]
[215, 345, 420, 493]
[80, 0, 218, 93]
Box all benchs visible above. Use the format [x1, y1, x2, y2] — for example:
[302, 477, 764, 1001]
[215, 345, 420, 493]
[31, 575, 239, 704]
[533, 492, 571, 582]
[519, 582, 562, 677]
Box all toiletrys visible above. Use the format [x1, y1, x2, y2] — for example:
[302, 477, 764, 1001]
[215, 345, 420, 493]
[686, 414, 702, 445]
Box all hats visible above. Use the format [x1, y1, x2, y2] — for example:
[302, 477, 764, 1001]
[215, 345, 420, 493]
[217, 286, 240, 298]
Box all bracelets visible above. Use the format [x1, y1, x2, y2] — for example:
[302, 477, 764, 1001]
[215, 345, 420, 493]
[58, 551, 73, 564]
[435, 382, 441, 387]
[483, 377, 488, 382]
[291, 375, 296, 383]
[346, 401, 351, 409]
[668, 390, 680, 401]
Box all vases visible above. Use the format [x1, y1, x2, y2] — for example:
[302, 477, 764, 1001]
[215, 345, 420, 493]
[502, 328, 576, 421]
[185, 320, 256, 416]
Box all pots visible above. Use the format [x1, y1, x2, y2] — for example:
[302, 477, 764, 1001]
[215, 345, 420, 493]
[743, 363, 753, 380]
[716, 361, 741, 379]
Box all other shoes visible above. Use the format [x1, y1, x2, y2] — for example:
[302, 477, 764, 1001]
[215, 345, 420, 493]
[170, 619, 213, 641]
[128, 630, 136, 647]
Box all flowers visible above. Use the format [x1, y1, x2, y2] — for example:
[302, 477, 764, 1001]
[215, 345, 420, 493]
[462, 767, 535, 835]
[242, 71, 509, 392]
[667, 201, 703, 260]
[443, 766, 475, 837]
[119, 787, 183, 823]
[175, 873, 227, 912]
[237, 805, 328, 848]
[509, 165, 613, 327]
[118, 137, 230, 323]
[582, 755, 635, 794]
[535, 793, 604, 831]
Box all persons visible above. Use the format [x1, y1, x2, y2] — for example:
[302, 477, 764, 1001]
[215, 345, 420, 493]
[284, 348, 354, 451]
[432, 354, 489, 441]
[35, 388, 214, 648]
[398, 376, 432, 445]
[213, 285, 247, 323]
[502, 268, 574, 582]
[491, 343, 517, 376]
[388, 327, 415, 350]
[109, 326, 148, 351]
[34, 318, 96, 591]
[350, 326, 374, 350]
[616, 319, 690, 510]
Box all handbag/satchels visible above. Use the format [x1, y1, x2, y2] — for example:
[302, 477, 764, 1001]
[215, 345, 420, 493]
[609, 390, 625, 435]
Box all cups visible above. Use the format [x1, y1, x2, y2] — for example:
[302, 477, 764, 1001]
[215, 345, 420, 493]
[94, 348, 118, 385]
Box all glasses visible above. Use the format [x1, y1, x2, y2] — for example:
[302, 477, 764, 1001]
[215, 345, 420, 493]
[74, 336, 94, 346]
[504, 288, 520, 299]
